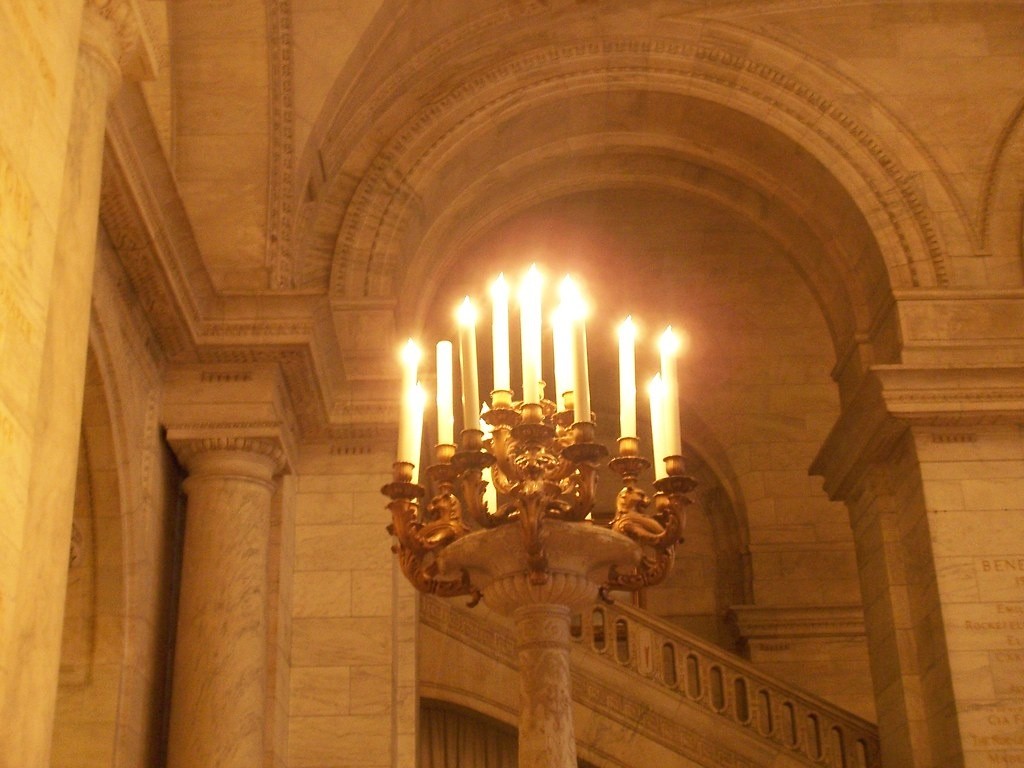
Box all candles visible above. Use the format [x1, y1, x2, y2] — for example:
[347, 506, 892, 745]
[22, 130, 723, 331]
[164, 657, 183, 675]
[388, 263, 685, 519]
